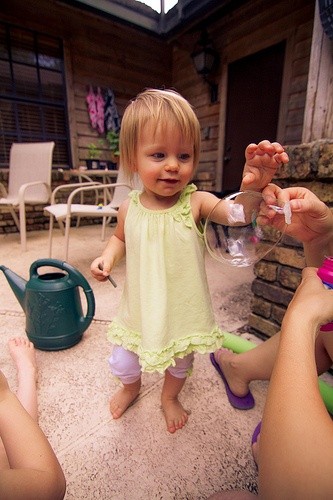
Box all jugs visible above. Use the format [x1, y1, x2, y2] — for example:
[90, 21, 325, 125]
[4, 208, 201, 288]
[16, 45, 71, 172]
[0, 257, 95, 351]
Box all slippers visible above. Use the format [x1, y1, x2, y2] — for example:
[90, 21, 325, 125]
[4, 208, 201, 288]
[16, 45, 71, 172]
[210, 352, 255, 410]
[252, 421, 261, 471]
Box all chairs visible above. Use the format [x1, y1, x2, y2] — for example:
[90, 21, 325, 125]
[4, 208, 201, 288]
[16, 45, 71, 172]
[42, 156, 133, 265]
[0, 141, 66, 253]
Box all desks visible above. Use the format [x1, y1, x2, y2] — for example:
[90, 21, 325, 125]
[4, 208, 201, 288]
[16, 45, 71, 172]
[61, 167, 120, 242]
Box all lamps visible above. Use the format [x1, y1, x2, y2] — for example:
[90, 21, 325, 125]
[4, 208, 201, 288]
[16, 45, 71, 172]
[189, 28, 219, 103]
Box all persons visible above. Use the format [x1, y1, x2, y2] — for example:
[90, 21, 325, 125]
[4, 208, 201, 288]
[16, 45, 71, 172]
[90, 89, 289, 434]
[1, 338, 66, 500]
[209, 184, 333, 500]
